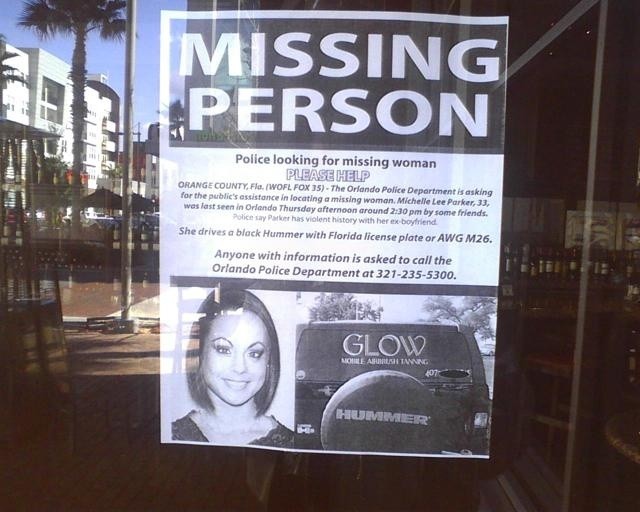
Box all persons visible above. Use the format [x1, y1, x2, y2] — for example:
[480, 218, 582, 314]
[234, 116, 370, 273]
[171, 287, 299, 451]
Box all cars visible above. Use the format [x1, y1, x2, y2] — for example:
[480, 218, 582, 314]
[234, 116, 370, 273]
[3, 206, 160, 245]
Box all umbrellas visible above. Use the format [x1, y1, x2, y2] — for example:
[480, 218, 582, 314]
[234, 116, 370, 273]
[80, 186, 123, 209]
[131, 193, 156, 212]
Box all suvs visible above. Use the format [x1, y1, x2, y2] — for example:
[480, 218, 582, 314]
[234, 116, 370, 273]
[294, 319, 490, 456]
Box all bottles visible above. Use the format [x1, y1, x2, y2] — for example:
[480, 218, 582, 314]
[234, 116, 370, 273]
[1, 222, 159, 311]
[504, 240, 640, 281]
